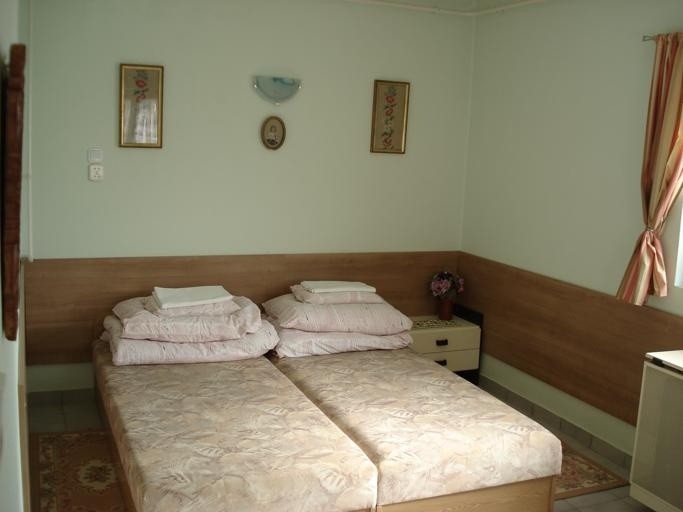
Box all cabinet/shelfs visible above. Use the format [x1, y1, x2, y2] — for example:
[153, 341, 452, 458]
[629, 351, 683, 512]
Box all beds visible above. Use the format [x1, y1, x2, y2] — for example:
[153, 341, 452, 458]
[92, 337, 377, 512]
[267, 349, 561, 512]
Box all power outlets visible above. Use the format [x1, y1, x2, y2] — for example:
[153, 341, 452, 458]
[89, 165, 103, 181]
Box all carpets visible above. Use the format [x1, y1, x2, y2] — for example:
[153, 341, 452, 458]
[30, 427, 630, 512]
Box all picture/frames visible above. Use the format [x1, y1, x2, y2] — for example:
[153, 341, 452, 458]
[369, 79, 410, 154]
[261, 116, 285, 150]
[120, 64, 163, 148]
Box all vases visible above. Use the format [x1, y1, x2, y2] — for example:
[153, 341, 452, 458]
[438, 300, 453, 320]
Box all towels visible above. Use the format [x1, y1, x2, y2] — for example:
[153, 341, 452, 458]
[300, 280, 377, 294]
[152, 285, 234, 308]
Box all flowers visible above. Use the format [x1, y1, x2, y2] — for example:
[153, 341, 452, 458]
[431, 271, 464, 299]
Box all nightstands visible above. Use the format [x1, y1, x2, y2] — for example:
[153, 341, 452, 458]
[408, 315, 481, 387]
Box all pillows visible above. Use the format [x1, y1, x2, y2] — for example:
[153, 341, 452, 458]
[290, 283, 383, 305]
[112, 293, 262, 343]
[145, 295, 239, 318]
[262, 292, 414, 336]
